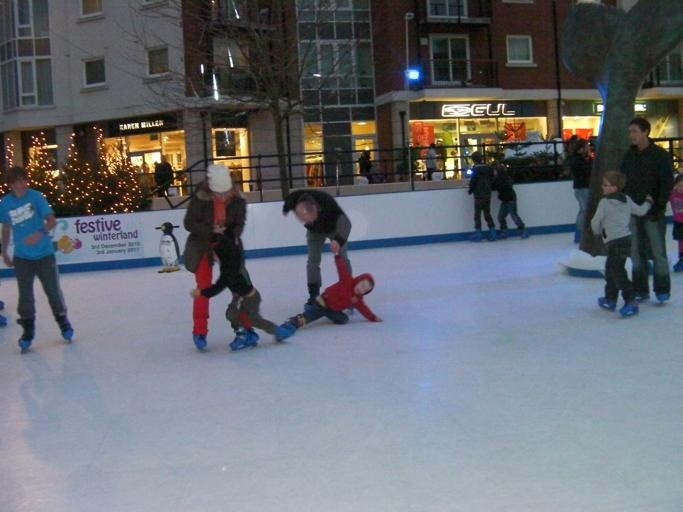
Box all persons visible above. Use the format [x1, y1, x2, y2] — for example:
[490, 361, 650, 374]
[0, 167, 74, 353]
[619, 117, 675, 306]
[282, 189, 351, 304]
[0, 299, 8, 328]
[276, 248, 383, 342]
[590, 171, 654, 318]
[426, 143, 441, 181]
[155, 155, 174, 198]
[670, 174, 683, 273]
[468, 151, 496, 241]
[570, 138, 594, 244]
[184, 164, 247, 351]
[360, 150, 373, 183]
[190, 225, 296, 347]
[491, 167, 529, 239]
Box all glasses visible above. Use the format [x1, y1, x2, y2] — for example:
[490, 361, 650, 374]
[603, 183, 614, 187]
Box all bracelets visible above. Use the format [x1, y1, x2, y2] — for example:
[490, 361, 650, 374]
[37, 228, 48, 236]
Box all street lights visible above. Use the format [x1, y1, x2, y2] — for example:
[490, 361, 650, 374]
[404, 14, 420, 148]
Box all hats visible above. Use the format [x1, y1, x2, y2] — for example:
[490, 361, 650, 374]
[205, 162, 231, 196]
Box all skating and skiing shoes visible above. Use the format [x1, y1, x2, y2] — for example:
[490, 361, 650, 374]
[486, 228, 496, 241]
[19, 338, 33, 354]
[0, 300, 7, 329]
[520, 230, 530, 239]
[597, 296, 616, 311]
[61, 328, 73, 342]
[619, 303, 639, 318]
[467, 230, 483, 242]
[656, 293, 669, 306]
[228, 330, 260, 351]
[275, 318, 294, 342]
[673, 257, 683, 272]
[192, 332, 207, 354]
[574, 228, 583, 243]
[496, 230, 508, 239]
[634, 294, 650, 304]
